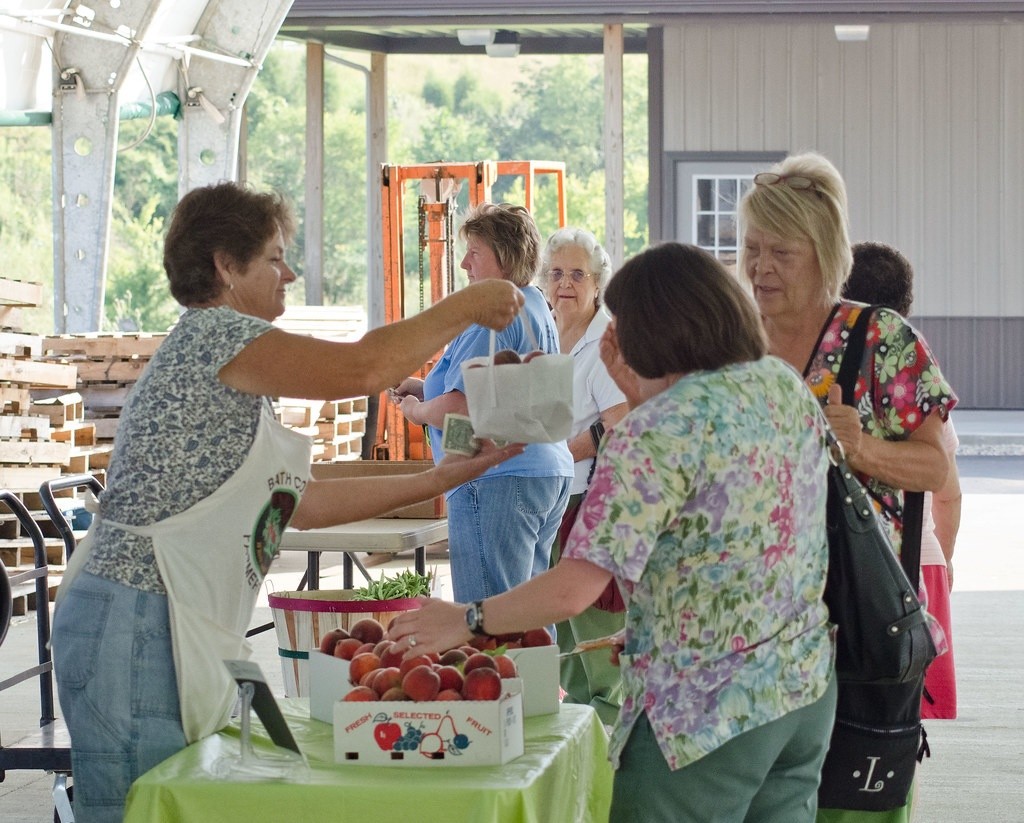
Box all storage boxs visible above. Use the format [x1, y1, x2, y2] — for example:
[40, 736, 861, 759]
[334, 677, 525, 767]
[309, 460, 448, 518]
[503, 643, 561, 717]
[308, 649, 353, 726]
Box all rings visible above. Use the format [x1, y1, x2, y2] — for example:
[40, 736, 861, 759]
[408, 634, 416, 647]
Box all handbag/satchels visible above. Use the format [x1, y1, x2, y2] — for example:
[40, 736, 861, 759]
[814, 673, 930, 813]
[460, 307, 575, 443]
[779, 356, 939, 688]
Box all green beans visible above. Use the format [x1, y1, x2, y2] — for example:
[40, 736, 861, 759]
[349, 566, 433, 601]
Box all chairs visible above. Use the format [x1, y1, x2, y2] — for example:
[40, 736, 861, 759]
[0, 476, 106, 823]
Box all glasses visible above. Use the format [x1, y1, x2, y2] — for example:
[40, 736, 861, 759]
[544, 270, 597, 282]
[753, 172, 822, 198]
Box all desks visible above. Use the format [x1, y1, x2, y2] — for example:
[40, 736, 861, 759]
[246, 515, 449, 640]
[121, 698, 615, 823]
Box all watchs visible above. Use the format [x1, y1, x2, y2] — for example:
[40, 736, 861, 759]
[464, 600, 493, 637]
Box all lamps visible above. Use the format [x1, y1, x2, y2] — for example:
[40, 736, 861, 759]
[458, 29, 496, 46]
[834, 24, 871, 41]
[483, 32, 522, 57]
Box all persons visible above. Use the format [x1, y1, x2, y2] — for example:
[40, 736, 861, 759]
[394, 151, 962, 823]
[48, 181, 528, 823]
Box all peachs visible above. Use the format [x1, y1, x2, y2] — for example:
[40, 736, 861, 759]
[320, 619, 551, 702]
[468, 349, 547, 367]
